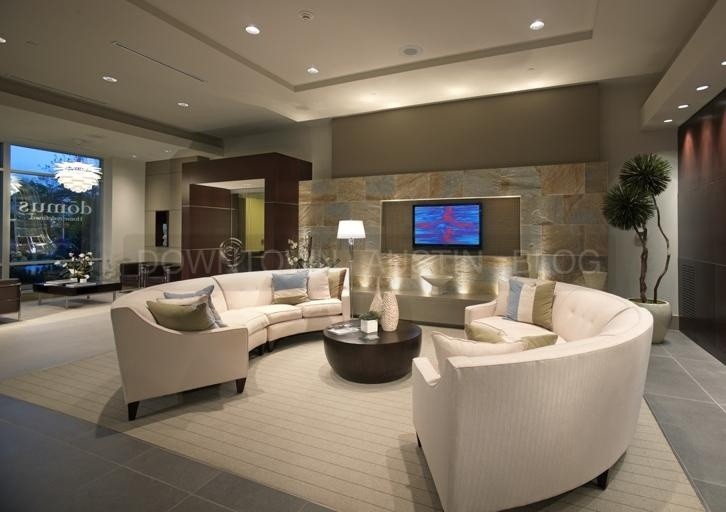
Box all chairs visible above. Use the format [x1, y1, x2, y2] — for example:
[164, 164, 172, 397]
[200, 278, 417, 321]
[0, 277, 24, 322]
[141, 261, 181, 288]
[116, 262, 142, 293]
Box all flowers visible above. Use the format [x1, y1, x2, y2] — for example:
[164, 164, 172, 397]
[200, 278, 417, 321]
[53, 251, 95, 278]
[281, 230, 340, 268]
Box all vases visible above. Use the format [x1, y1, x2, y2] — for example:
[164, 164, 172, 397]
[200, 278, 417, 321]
[69, 275, 87, 283]
[368, 287, 382, 327]
[380, 290, 401, 332]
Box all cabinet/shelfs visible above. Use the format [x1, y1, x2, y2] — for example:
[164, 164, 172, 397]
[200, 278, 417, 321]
[350, 284, 493, 328]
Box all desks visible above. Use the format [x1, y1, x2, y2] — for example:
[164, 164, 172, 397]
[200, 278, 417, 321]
[30, 279, 122, 310]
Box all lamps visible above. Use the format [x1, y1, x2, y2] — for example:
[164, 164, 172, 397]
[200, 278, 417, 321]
[336, 217, 369, 259]
[51, 135, 103, 195]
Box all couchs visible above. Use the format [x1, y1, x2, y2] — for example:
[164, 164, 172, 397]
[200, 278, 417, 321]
[106, 264, 353, 422]
[407, 272, 660, 511]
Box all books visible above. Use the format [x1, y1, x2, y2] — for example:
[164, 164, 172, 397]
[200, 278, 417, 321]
[328, 324, 359, 336]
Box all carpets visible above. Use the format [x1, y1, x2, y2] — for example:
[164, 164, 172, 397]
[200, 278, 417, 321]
[0, 346, 709, 512]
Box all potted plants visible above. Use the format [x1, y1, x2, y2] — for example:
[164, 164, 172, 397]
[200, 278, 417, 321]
[357, 310, 380, 334]
[599, 149, 676, 345]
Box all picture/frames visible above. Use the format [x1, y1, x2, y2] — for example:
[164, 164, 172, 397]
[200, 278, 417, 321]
[155, 210, 169, 248]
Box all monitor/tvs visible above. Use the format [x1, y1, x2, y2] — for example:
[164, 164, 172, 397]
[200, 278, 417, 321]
[413, 203, 482, 249]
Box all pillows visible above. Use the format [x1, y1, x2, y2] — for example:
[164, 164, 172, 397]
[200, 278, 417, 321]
[493, 277, 510, 318]
[327, 268, 348, 300]
[162, 284, 228, 327]
[504, 275, 562, 333]
[271, 268, 310, 304]
[422, 323, 529, 377]
[306, 266, 335, 301]
[155, 292, 216, 322]
[145, 299, 216, 331]
[463, 318, 561, 352]
